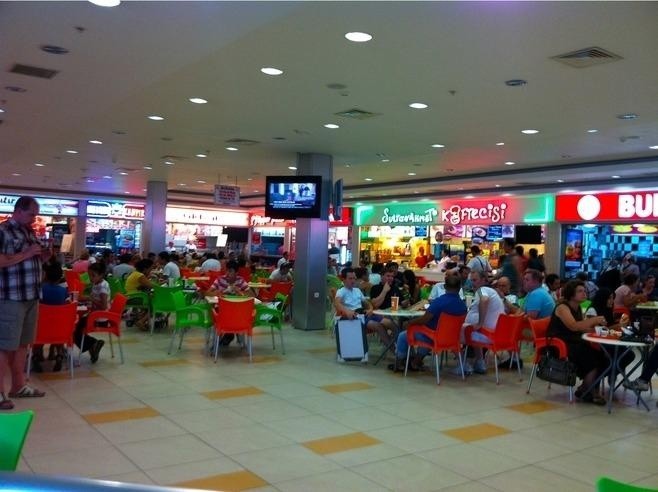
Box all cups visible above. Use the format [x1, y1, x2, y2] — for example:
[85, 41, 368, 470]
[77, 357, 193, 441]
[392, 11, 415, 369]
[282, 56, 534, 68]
[366, 244, 368, 250]
[168, 277, 175, 288]
[183, 279, 189, 288]
[391, 296, 399, 310]
[465, 295, 472, 305]
[601, 322, 635, 337]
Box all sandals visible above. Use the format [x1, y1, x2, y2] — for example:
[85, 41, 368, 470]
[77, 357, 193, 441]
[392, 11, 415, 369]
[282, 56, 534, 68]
[575, 384, 606, 405]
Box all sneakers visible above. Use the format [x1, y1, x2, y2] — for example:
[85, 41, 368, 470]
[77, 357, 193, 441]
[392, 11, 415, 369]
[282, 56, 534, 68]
[498, 359, 522, 369]
[90, 340, 104, 362]
[474, 360, 486, 373]
[448, 360, 473, 375]
[136, 319, 147, 330]
[623, 379, 648, 391]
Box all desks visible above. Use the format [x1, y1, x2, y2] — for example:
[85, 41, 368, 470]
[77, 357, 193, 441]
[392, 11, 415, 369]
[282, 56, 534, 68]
[370, 309, 427, 375]
[578, 329, 653, 416]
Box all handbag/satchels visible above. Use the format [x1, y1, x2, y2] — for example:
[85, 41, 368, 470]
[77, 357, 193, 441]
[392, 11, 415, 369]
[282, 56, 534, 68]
[537, 355, 576, 386]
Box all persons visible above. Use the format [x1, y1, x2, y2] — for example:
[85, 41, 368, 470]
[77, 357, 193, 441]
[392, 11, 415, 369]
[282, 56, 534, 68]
[31, 237, 294, 373]
[329, 238, 658, 405]
[0, 195, 54, 410]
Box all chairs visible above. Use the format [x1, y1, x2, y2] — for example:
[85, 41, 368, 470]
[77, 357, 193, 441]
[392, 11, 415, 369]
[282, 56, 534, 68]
[594, 474, 656, 492]
[403, 311, 465, 385]
[0, 408, 35, 472]
[148, 281, 184, 335]
[166, 289, 210, 357]
[526, 315, 575, 403]
[0, 275, 297, 326]
[327, 258, 657, 342]
[76, 292, 127, 366]
[23, 300, 79, 381]
[210, 296, 255, 365]
[464, 311, 527, 386]
[252, 291, 289, 359]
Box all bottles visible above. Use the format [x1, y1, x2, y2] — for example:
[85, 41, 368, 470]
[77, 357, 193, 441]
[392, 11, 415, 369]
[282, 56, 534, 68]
[70, 290, 79, 303]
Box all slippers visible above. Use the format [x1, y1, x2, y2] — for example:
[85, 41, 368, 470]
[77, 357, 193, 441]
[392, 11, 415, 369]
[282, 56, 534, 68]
[388, 364, 405, 371]
[0, 385, 13, 409]
[8, 384, 44, 397]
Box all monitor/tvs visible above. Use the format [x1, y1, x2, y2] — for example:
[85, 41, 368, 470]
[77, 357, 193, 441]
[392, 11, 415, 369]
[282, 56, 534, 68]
[265, 176, 322, 217]
[223, 227, 248, 243]
[334, 178, 343, 220]
[515, 226, 541, 244]
